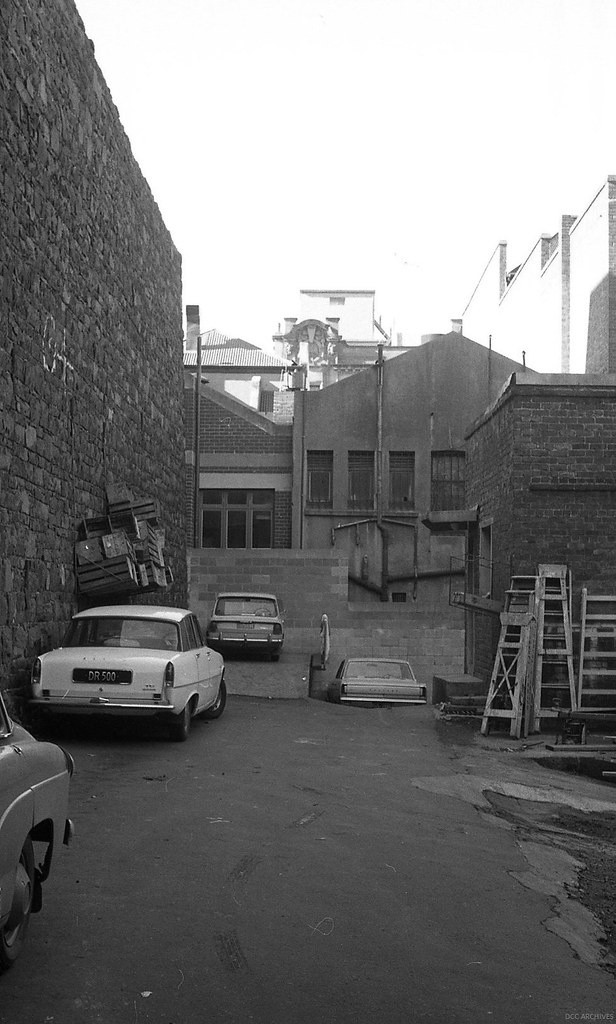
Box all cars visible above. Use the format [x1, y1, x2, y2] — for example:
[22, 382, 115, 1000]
[0, 694, 77, 976]
[325, 658, 429, 707]
[206, 591, 287, 662]
[25, 605, 227, 742]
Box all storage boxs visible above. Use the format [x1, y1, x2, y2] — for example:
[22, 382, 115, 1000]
[74, 481, 174, 598]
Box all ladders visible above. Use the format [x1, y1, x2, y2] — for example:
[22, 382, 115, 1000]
[479, 563, 615, 739]
